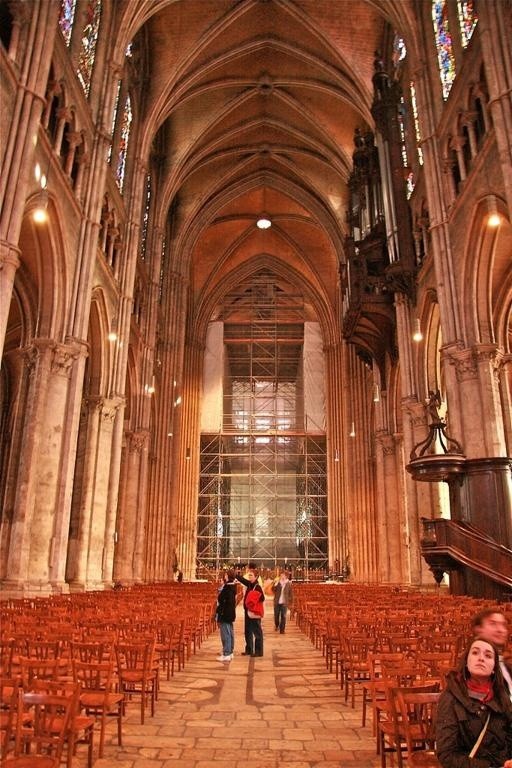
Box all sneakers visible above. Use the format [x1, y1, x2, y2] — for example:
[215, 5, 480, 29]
[275, 625, 285, 634]
[216, 650, 233, 661]
[242, 652, 262, 657]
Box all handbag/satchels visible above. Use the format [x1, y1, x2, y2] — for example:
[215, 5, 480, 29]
[247, 610, 261, 619]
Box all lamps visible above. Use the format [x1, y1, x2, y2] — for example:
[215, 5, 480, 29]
[256, 158, 272, 229]
[413, 318, 422, 341]
[185, 448, 190, 460]
[373, 385, 379, 402]
[33, 118, 59, 222]
[108, 320, 117, 340]
[349, 422, 355, 437]
[475, 114, 501, 226]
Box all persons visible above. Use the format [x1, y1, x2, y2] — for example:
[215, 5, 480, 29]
[216, 570, 236, 662]
[434, 638, 512, 768]
[471, 609, 511, 702]
[272, 572, 294, 635]
[235, 570, 264, 657]
